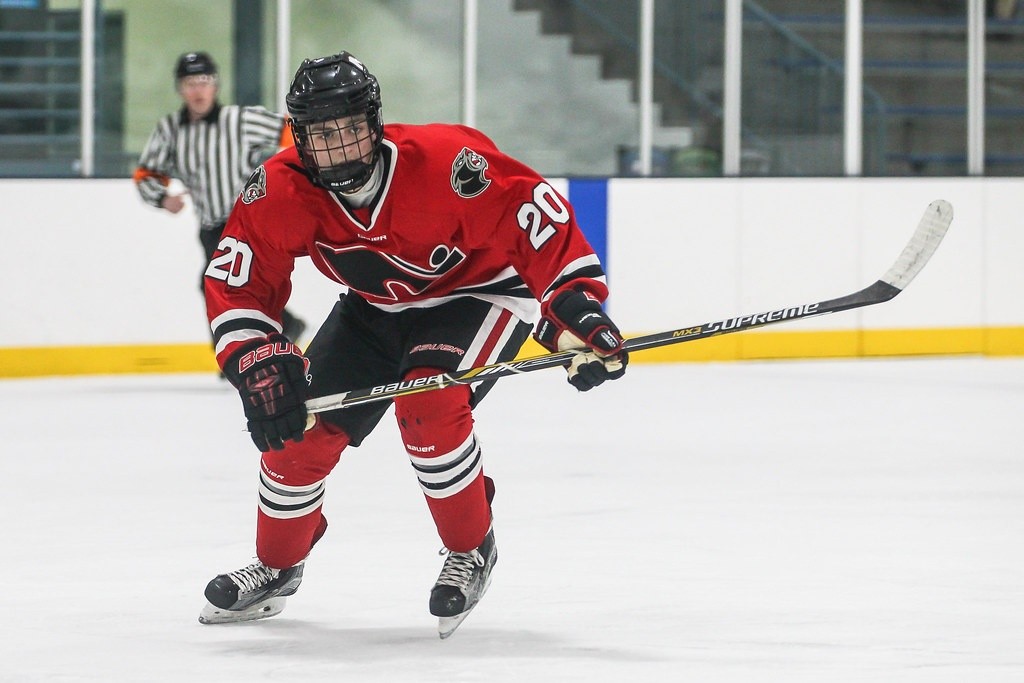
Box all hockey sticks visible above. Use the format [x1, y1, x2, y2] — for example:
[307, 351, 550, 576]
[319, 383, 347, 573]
[302, 192, 960, 415]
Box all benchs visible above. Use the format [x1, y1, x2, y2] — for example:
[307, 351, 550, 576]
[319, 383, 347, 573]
[0, 0, 78, 155]
[704, 0, 1024, 176]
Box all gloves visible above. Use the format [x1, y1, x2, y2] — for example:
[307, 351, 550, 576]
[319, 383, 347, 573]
[223, 332, 309, 452]
[533, 288, 628, 392]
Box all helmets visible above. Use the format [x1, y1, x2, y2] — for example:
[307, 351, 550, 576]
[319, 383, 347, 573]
[285, 51, 384, 192]
[175, 52, 213, 79]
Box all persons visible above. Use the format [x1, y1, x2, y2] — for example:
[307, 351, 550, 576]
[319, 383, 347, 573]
[198, 52, 630, 640]
[134, 51, 307, 379]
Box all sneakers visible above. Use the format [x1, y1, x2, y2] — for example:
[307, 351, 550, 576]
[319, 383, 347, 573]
[429, 474, 498, 639]
[199, 511, 329, 625]
